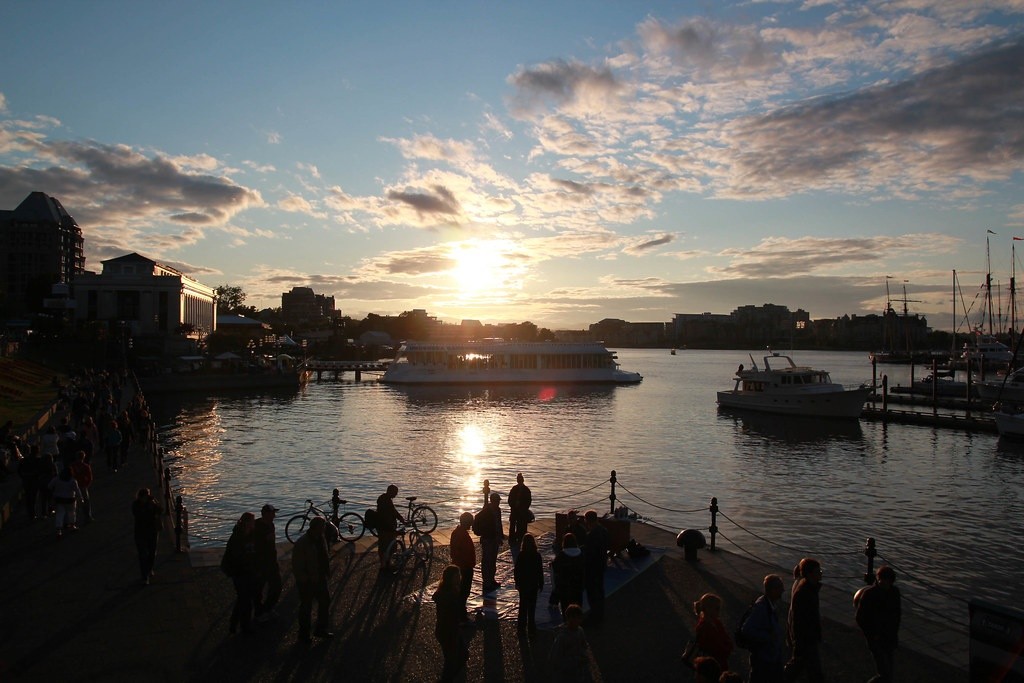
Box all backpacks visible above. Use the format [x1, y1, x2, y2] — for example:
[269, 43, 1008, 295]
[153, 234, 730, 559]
[472, 506, 500, 537]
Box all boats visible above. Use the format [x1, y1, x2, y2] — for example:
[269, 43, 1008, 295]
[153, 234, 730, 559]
[715, 346, 875, 419]
[992, 399, 1024, 436]
[374, 338, 645, 384]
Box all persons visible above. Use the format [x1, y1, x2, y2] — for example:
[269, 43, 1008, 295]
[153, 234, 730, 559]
[546, 603, 597, 683]
[548, 532, 584, 640]
[377, 485, 406, 568]
[132, 488, 164, 585]
[450, 513, 476, 623]
[221, 512, 258, 633]
[0, 364, 155, 541]
[563, 511, 608, 627]
[692, 657, 744, 683]
[514, 534, 544, 639]
[293, 518, 335, 643]
[508, 475, 532, 545]
[693, 592, 734, 673]
[254, 504, 280, 622]
[741, 574, 788, 683]
[480, 494, 501, 592]
[432, 566, 475, 683]
[783, 558, 828, 683]
[855, 566, 902, 683]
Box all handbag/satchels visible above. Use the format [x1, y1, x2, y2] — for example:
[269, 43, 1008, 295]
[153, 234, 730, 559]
[735, 595, 773, 649]
[680, 641, 709, 668]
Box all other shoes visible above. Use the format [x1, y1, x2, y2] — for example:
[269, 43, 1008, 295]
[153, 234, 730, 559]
[315, 632, 335, 638]
[298, 636, 315, 643]
[65, 524, 80, 531]
[144, 567, 154, 586]
[57, 529, 62, 537]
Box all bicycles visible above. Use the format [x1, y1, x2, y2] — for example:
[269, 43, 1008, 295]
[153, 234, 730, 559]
[383, 519, 433, 576]
[369, 496, 438, 534]
[285, 499, 366, 544]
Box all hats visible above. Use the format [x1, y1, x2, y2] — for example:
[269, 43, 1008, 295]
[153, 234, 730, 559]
[261, 504, 280, 512]
[490, 494, 501, 501]
[584, 511, 598, 521]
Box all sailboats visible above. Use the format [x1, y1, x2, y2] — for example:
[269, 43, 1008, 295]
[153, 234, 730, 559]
[869, 229, 1024, 402]
